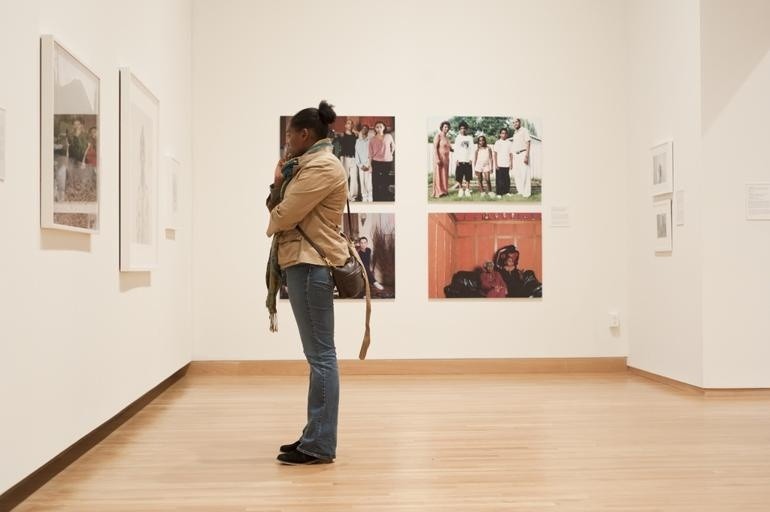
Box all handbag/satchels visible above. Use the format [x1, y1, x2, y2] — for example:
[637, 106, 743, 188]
[332, 256, 368, 300]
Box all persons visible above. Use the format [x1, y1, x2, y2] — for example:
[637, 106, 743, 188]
[265, 100, 348, 465]
[499, 256, 523, 291]
[333, 119, 395, 202]
[59, 119, 96, 167]
[430, 118, 532, 198]
[357, 237, 384, 292]
[480, 261, 508, 298]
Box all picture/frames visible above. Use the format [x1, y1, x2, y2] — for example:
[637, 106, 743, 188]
[646, 140, 674, 196]
[650, 199, 673, 253]
[120, 66, 162, 272]
[40, 34, 104, 237]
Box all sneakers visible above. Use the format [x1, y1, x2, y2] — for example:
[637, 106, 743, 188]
[279, 440, 300, 452]
[276, 452, 325, 465]
[457, 187, 470, 197]
[373, 281, 385, 291]
[479, 191, 512, 199]
